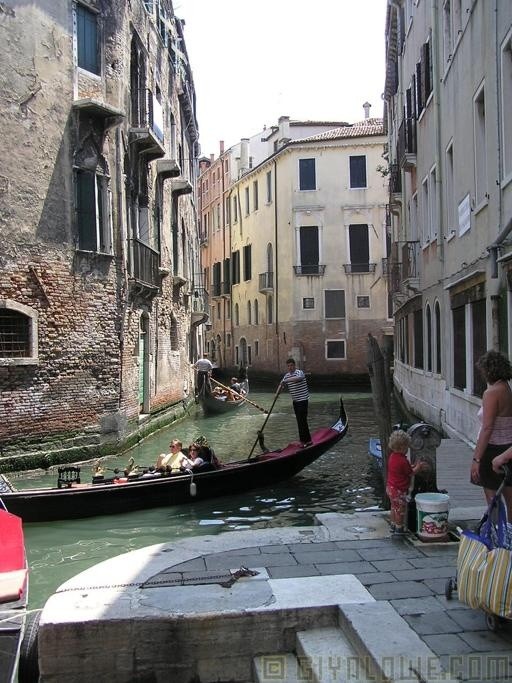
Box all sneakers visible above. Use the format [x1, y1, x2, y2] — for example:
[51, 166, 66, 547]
[390, 523, 411, 535]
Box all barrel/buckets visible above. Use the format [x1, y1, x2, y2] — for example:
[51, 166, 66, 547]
[415, 493, 450, 538]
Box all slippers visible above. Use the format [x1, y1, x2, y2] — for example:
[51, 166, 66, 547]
[299, 441, 312, 448]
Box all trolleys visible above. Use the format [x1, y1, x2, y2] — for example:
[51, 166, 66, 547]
[437, 459, 512, 631]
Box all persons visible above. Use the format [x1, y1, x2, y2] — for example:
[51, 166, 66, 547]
[155, 438, 188, 469]
[491, 444, 512, 475]
[282, 358, 313, 449]
[468, 346, 512, 530]
[193, 351, 213, 396]
[230, 376, 239, 392]
[385, 429, 427, 535]
[178, 442, 204, 469]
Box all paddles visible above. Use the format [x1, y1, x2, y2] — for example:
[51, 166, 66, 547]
[192, 368, 269, 414]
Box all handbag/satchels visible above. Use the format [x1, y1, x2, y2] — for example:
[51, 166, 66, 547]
[457, 531, 512, 620]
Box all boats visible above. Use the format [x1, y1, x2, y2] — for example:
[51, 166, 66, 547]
[369, 419, 408, 474]
[0, 494, 32, 682]
[194, 364, 252, 413]
[0, 394, 352, 526]
[211, 362, 220, 376]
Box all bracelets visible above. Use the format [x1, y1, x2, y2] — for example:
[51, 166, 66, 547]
[472, 456, 482, 463]
